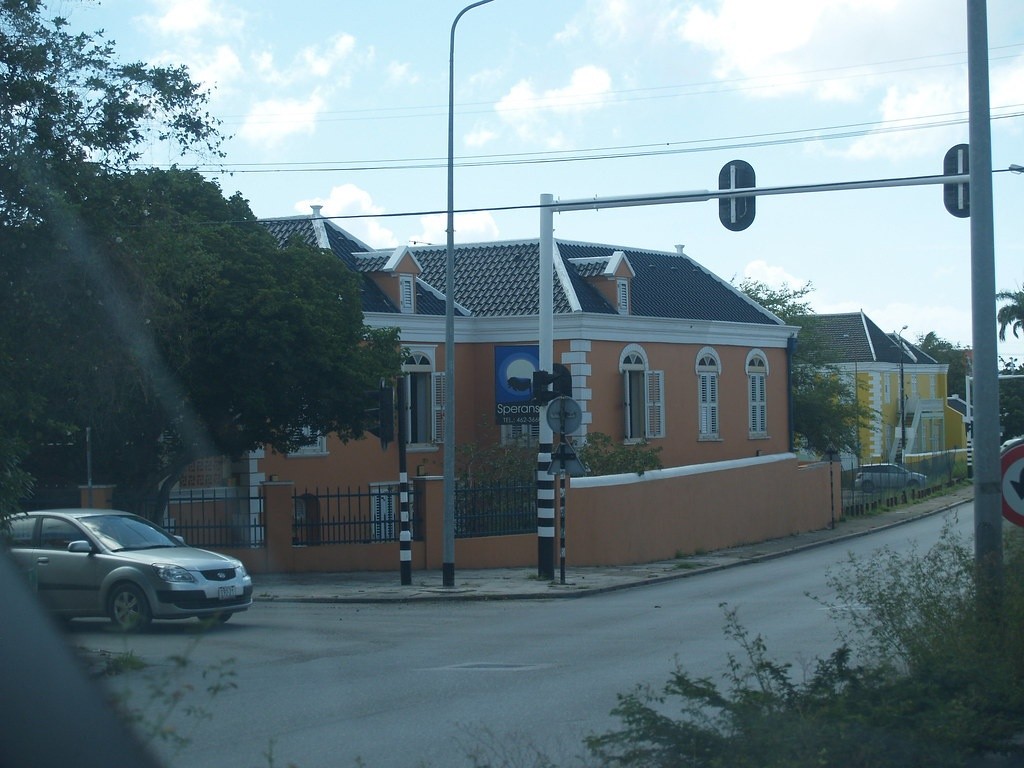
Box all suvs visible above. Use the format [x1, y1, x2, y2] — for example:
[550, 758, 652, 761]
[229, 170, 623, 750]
[854, 463, 927, 493]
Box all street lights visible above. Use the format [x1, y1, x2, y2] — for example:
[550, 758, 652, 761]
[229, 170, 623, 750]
[898, 325, 909, 450]
[844, 334, 861, 464]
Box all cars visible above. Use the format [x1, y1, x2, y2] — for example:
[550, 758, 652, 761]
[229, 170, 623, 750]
[0, 508, 253, 634]
